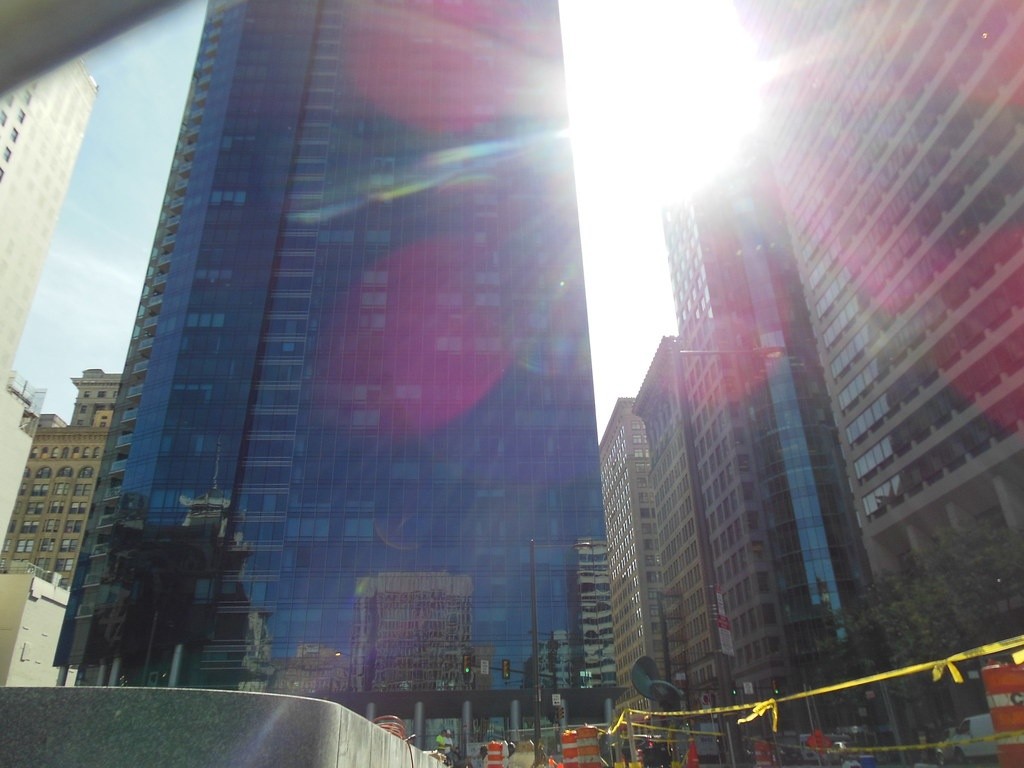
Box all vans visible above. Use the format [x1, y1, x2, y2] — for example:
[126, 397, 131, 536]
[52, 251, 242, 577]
[799, 734, 851, 760]
[951, 714, 998, 763]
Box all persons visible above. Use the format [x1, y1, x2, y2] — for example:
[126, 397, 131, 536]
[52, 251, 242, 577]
[507, 738, 515, 756]
[436, 729, 453, 755]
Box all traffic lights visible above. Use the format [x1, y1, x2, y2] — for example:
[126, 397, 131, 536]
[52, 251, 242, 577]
[463, 656, 470, 673]
[559, 707, 564, 718]
[502, 660, 509, 679]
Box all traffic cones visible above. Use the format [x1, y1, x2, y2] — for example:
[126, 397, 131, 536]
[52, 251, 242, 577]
[688, 740, 700, 768]
[548, 756, 554, 768]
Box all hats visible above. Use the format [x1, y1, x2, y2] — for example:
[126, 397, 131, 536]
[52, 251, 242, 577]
[447, 730, 450, 734]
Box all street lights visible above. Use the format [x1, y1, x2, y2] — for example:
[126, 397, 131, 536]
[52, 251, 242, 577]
[336, 652, 352, 688]
[528, 538, 592, 765]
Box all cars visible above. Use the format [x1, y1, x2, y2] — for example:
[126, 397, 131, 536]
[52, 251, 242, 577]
[827, 742, 860, 762]
[608, 735, 671, 761]
[935, 727, 956, 766]
